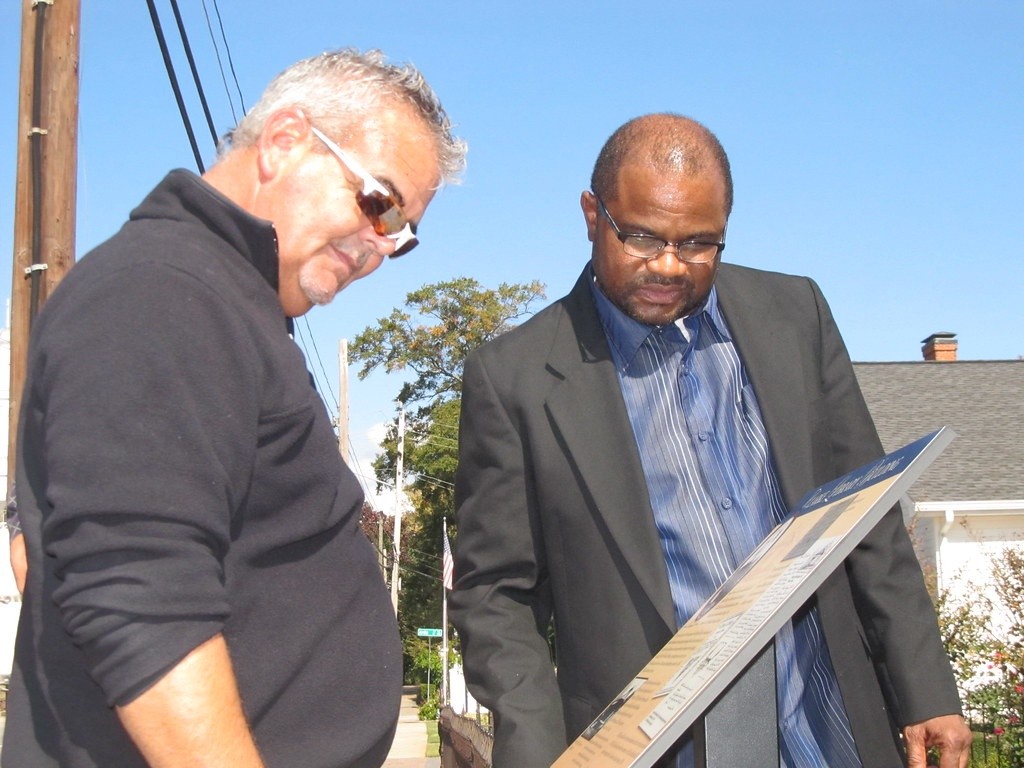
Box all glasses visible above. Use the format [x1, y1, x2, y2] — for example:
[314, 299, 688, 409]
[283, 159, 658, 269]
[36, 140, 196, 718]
[595, 197, 726, 263]
[310, 126, 418, 260]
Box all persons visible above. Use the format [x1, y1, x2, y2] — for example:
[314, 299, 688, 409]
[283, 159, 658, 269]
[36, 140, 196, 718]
[449, 114, 972, 768]
[0, 46, 467, 768]
